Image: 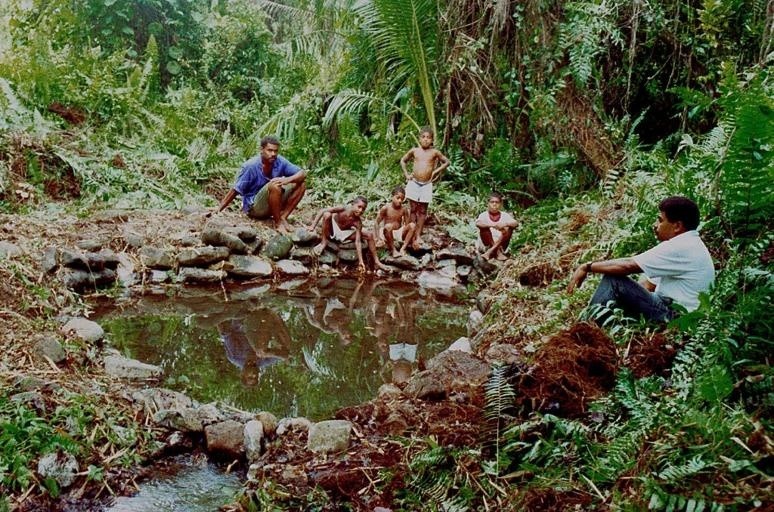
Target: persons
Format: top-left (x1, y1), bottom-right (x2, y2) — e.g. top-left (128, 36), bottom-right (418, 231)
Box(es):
top-left (365, 292), bottom-right (404, 356)
top-left (376, 186), bottom-right (416, 258)
top-left (561, 195), bottom-right (717, 336)
top-left (400, 126), bottom-right (450, 246)
top-left (379, 298), bottom-right (427, 384)
top-left (304, 274), bottom-right (365, 346)
top-left (306, 196), bottom-right (391, 273)
top-left (475, 191), bottom-right (519, 262)
top-left (216, 311), bottom-right (292, 386)
top-left (209, 135), bottom-right (306, 236)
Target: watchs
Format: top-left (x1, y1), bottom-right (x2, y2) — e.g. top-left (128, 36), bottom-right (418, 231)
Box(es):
top-left (585, 261), bottom-right (594, 274)
